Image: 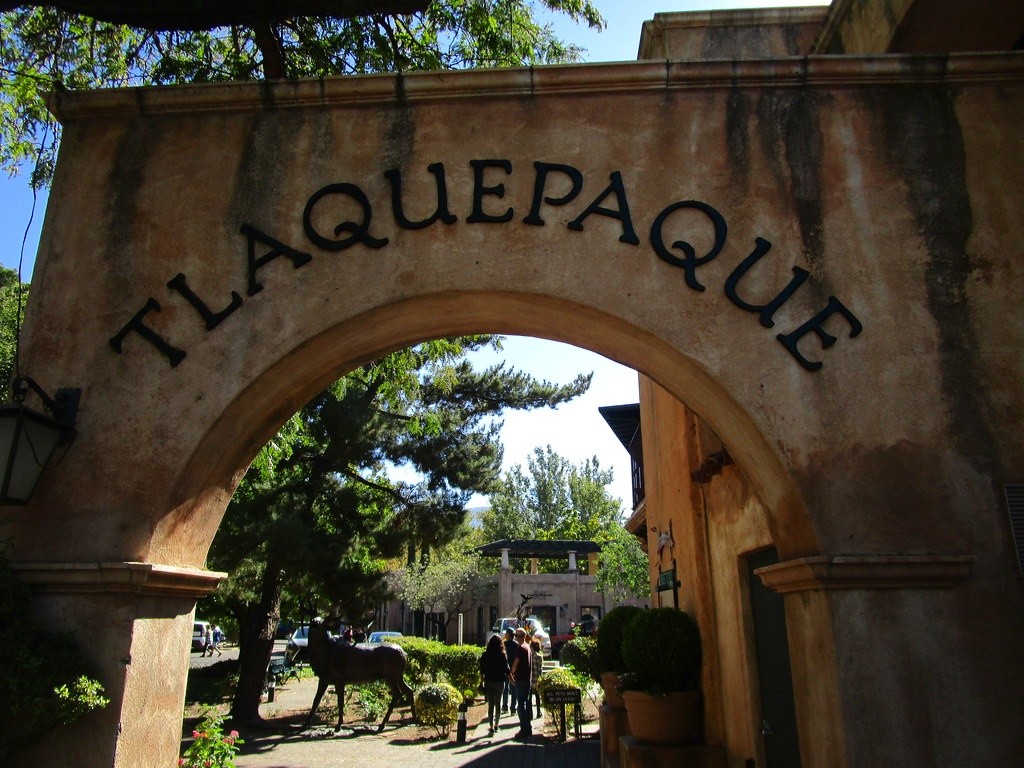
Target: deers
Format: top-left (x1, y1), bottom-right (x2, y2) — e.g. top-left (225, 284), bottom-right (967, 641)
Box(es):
top-left (298, 591), bottom-right (420, 732)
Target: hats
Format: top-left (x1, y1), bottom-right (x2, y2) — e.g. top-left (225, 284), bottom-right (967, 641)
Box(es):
top-left (507, 627), bottom-right (516, 634)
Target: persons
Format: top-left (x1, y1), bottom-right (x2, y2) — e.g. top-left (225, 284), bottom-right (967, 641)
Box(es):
top-left (343, 624), bottom-right (372, 644)
top-left (480, 627), bottom-right (543, 739)
top-left (201, 624), bottom-right (223, 658)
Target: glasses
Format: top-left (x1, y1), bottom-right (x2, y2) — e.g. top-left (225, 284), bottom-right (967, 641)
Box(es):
top-left (514, 633), bottom-right (525, 638)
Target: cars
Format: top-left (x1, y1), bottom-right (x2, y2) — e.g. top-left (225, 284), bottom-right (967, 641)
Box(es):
top-left (283, 626), bottom-right (339, 667)
top-left (192, 621), bottom-right (226, 653)
top-left (367, 630), bottom-right (403, 645)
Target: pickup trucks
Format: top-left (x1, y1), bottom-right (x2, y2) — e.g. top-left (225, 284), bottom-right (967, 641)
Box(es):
top-left (485, 618), bottom-right (552, 659)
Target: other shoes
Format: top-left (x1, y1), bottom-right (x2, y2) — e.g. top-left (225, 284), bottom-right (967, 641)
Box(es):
top-left (537, 712), bottom-right (542, 718)
top-left (494, 726), bottom-right (498, 733)
top-left (502, 705), bottom-right (508, 714)
top-left (515, 730), bottom-right (532, 740)
top-left (488, 728), bottom-right (494, 736)
top-left (510, 708), bottom-right (516, 716)
